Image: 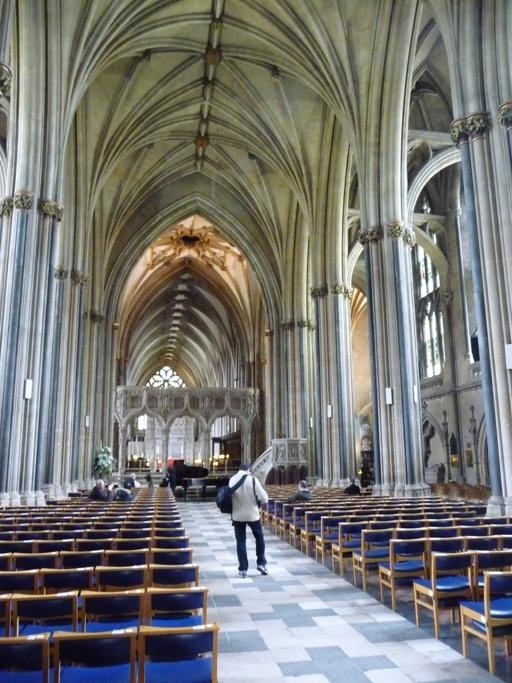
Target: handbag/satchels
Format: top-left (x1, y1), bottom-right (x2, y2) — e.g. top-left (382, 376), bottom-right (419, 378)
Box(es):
top-left (216, 474), bottom-right (248, 513)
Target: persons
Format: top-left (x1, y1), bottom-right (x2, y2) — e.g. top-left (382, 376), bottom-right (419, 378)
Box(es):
top-left (228, 464), bottom-right (269, 578)
top-left (145, 473), bottom-right (153, 488)
top-left (128, 473), bottom-right (140, 488)
top-left (89, 478), bottom-right (109, 500)
top-left (288, 480), bottom-right (312, 504)
top-left (162, 473), bottom-right (169, 487)
top-left (113, 488), bottom-right (134, 501)
top-left (344, 478), bottom-right (360, 495)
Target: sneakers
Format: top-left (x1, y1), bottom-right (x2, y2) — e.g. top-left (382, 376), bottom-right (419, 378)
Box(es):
top-left (238, 570), bottom-right (247, 578)
top-left (257, 564), bottom-right (268, 575)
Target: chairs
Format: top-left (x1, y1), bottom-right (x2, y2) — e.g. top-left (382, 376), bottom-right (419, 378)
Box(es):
top-left (498, 534), bottom-right (512, 573)
top-left (424, 536), bottom-right (465, 578)
top-left (80, 588), bottom-right (145, 631)
top-left (398, 520), bottom-right (427, 528)
top-left (428, 527), bottom-right (460, 538)
top-left (454, 518), bottom-right (482, 526)
top-left (10, 590), bottom-right (79, 637)
top-left (0, 552), bottom-right (13, 571)
top-left (149, 563), bottom-right (200, 588)
top-left (460, 569), bottom-right (512, 674)
top-left (279, 504), bottom-right (305, 542)
top-left (289, 507), bottom-right (317, 549)
top-left (413, 553), bottom-right (473, 640)
top-left (331, 521), bottom-right (369, 575)
top-left (138, 623), bottom-right (220, 683)
top-left (52, 627), bottom-right (138, 680)
top-left (32, 538), bottom-right (75, 551)
top-left (300, 510), bottom-right (329, 556)
top-left (39, 567), bottom-right (94, 593)
top-left (378, 538), bottom-right (427, 612)
top-left (0, 570), bottom-right (40, 594)
top-left (146, 587), bottom-right (208, 626)
top-left (314, 516), bottom-right (348, 565)
top-left (0, 487), bottom-right (185, 536)
top-left (96, 565), bottom-right (149, 591)
top-left (0, 541), bottom-right (32, 552)
top-left (461, 525), bottom-right (491, 536)
top-left (352, 528), bottom-right (394, 591)
top-left (151, 547), bottom-right (193, 564)
top-left (428, 519), bottom-right (453, 527)
top-left (476, 550), bottom-right (512, 601)
top-left (1, 593), bottom-right (11, 637)
top-left (395, 528), bottom-right (427, 562)
top-left (0, 634), bottom-right (49, 682)
top-left (369, 520), bottom-right (398, 529)
top-left (253, 494), bottom-right (278, 535)
top-left (76, 538), bottom-right (112, 549)
top-left (315, 493), bottom-right (487, 518)
top-left (105, 548), bottom-right (150, 565)
top-left (153, 535), bottom-right (192, 547)
top-left (482, 518), bottom-right (507, 524)
top-left (467, 536), bottom-right (500, 594)
top-left (60, 550), bottom-right (105, 568)
top-left (12, 552), bottom-right (59, 570)
top-left (491, 524), bottom-right (512, 536)
top-left (112, 536), bottom-right (153, 548)
